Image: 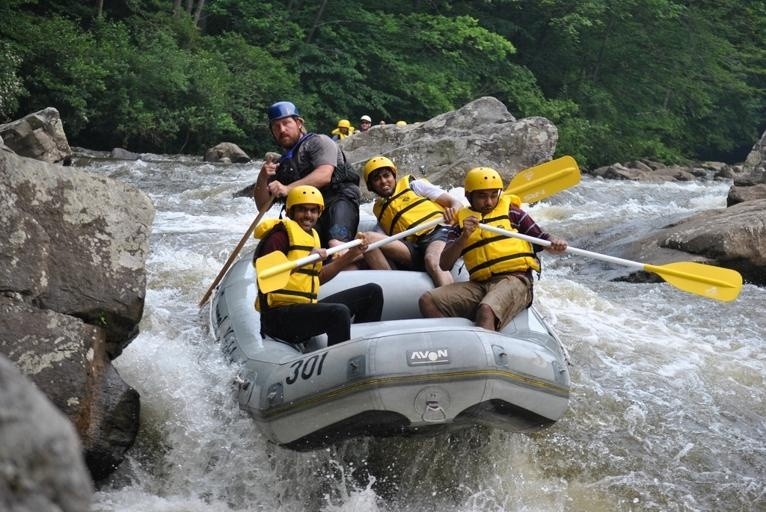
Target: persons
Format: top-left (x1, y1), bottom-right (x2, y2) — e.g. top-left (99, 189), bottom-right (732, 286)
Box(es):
top-left (252, 185), bottom-right (384, 348)
top-left (359, 156), bottom-right (464, 288)
top-left (332, 119), bottom-right (361, 141)
top-left (358, 114), bottom-right (372, 131)
top-left (254, 101), bottom-right (361, 243)
top-left (418, 167), bottom-right (567, 331)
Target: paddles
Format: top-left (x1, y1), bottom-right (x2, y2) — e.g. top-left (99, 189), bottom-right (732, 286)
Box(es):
top-left (364, 155), bottom-right (582, 254)
top-left (477, 221), bottom-right (744, 303)
top-left (255, 238), bottom-right (364, 293)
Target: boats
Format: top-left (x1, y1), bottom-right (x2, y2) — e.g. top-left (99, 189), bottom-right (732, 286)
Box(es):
top-left (209, 235), bottom-right (576, 453)
top-left (208, 201), bottom-right (571, 451)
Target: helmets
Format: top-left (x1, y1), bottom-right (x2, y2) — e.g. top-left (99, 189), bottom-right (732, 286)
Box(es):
top-left (364, 157), bottom-right (397, 195)
top-left (338, 120), bottom-right (351, 128)
top-left (360, 115), bottom-right (371, 123)
top-left (464, 167), bottom-right (503, 214)
top-left (267, 100), bottom-right (300, 122)
top-left (285, 184), bottom-right (325, 219)
